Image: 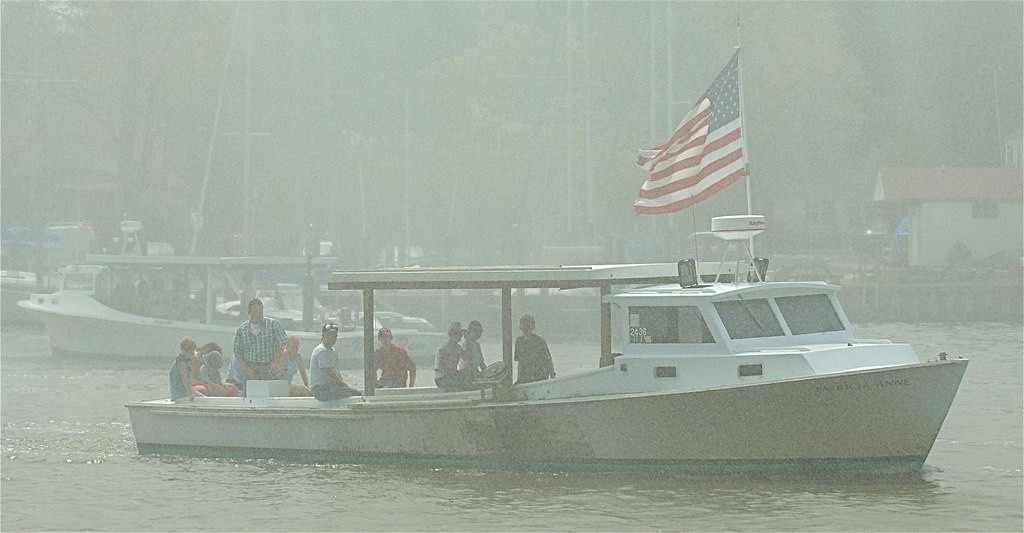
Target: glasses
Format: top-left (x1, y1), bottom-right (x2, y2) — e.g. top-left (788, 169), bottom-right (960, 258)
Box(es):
top-left (324, 324), bottom-right (338, 330)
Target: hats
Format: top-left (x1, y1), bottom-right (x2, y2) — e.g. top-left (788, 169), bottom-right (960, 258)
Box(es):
top-left (378, 328), bottom-right (392, 337)
top-left (202, 351), bottom-right (222, 369)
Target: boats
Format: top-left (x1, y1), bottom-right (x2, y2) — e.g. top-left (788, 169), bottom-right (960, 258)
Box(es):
top-left (124, 14), bottom-right (972, 483)
top-left (1, 5), bottom-right (452, 366)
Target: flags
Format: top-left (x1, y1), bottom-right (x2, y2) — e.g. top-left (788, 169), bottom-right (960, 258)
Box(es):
top-left (633, 48), bottom-right (749, 213)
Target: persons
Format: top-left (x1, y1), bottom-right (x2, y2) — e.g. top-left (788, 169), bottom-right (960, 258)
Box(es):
top-left (374, 328), bottom-right (416, 388)
top-left (311, 323), bottom-right (362, 402)
top-left (435, 320), bottom-right (486, 393)
top-left (514, 315), bottom-right (555, 384)
top-left (170, 299), bottom-right (311, 401)
